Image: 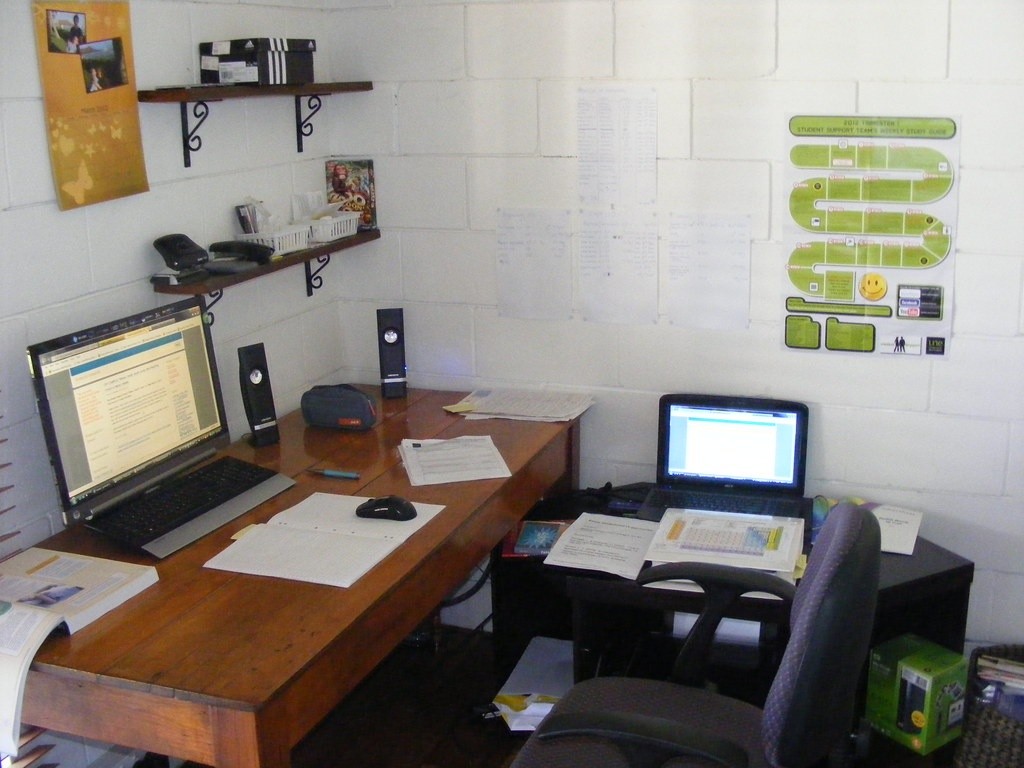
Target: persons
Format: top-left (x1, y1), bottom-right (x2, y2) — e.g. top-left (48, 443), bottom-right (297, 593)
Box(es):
top-left (65, 15), bottom-right (85, 54)
top-left (88, 66), bottom-right (110, 92)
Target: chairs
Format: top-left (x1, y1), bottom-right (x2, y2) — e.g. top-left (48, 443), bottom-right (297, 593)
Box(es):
top-left (514, 499), bottom-right (883, 768)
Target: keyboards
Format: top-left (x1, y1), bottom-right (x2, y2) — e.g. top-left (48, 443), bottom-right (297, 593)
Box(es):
top-left (82, 455), bottom-right (297, 559)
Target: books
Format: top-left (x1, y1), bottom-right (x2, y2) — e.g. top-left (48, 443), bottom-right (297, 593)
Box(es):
top-left (502, 520), bottom-right (575, 558)
top-left (977, 655), bottom-right (1024, 689)
top-left (202, 493), bottom-right (446, 588)
top-left (0, 546), bottom-right (160, 757)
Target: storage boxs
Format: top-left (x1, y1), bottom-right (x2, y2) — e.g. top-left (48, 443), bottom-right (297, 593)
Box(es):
top-left (864, 630), bottom-right (969, 756)
top-left (198, 37), bottom-right (316, 95)
top-left (326, 158), bottom-right (376, 232)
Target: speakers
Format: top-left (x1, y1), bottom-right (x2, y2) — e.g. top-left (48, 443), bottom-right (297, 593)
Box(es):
top-left (377, 307), bottom-right (407, 399)
top-left (238, 342), bottom-right (280, 449)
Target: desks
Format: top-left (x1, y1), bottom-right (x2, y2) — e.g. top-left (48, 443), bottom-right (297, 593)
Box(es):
top-left (1, 381), bottom-right (581, 768)
top-left (491, 481), bottom-right (976, 746)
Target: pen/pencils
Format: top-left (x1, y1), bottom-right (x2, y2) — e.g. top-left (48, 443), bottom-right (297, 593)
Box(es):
top-left (304, 468), bottom-right (361, 479)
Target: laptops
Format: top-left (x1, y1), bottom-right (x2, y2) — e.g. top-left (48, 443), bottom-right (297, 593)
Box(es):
top-left (635, 393), bottom-right (808, 521)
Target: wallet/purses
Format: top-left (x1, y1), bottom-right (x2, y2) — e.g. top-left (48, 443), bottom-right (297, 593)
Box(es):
top-left (301, 384), bottom-right (377, 431)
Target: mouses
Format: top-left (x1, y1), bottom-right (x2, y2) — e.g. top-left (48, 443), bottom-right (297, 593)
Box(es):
top-left (356, 494), bottom-right (418, 521)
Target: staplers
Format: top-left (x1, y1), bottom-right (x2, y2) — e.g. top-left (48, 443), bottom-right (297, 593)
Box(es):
top-left (210, 240), bottom-right (274, 265)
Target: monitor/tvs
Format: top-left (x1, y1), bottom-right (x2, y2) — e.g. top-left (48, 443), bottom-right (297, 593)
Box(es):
top-left (26, 295), bottom-right (231, 526)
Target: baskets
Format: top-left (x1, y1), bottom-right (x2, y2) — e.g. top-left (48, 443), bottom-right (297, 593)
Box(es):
top-left (951, 645), bottom-right (1024, 768)
